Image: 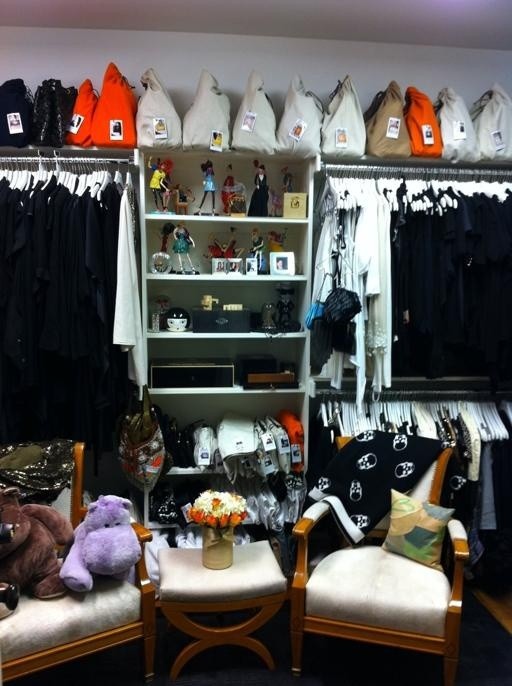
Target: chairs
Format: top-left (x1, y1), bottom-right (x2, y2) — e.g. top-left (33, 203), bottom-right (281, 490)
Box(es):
top-left (287, 435), bottom-right (470, 684)
top-left (1, 440), bottom-right (156, 683)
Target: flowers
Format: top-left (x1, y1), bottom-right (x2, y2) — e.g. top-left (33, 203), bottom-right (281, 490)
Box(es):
top-left (189, 489), bottom-right (248, 529)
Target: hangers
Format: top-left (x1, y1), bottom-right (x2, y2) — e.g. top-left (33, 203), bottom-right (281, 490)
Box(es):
top-left (0, 155), bottom-right (134, 201)
top-left (317, 384), bottom-right (512, 443)
top-left (315, 162), bottom-right (512, 213)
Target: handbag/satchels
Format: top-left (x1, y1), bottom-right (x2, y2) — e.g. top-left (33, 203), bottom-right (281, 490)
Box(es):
top-left (306, 273), bottom-right (362, 376)
top-left (64, 78), bottom-right (101, 149)
top-left (273, 73), bottom-right (324, 160)
top-left (91, 63), bottom-right (137, 149)
top-left (118, 385), bottom-right (167, 494)
top-left (469, 83), bottom-right (510, 161)
top-left (136, 68), bottom-right (182, 151)
top-left (433, 86), bottom-right (482, 162)
top-left (169, 410), bottom-right (304, 485)
top-left (231, 73), bottom-right (276, 155)
top-left (1, 78), bottom-right (35, 149)
top-left (403, 86), bottom-right (442, 158)
top-left (182, 68), bottom-right (231, 152)
top-left (364, 80), bottom-right (412, 159)
top-left (320, 75), bottom-right (366, 159)
top-left (31, 78), bottom-right (78, 149)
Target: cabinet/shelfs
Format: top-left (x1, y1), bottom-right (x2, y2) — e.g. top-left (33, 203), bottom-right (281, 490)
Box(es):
top-left (141, 151), bottom-right (315, 532)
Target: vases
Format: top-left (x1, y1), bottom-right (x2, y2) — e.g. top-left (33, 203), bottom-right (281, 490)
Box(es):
top-left (200, 528), bottom-right (234, 568)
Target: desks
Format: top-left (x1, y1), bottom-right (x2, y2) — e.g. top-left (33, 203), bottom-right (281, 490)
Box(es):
top-left (150, 543), bottom-right (286, 675)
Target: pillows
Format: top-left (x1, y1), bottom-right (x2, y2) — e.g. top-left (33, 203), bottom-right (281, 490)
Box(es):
top-left (383, 487), bottom-right (456, 573)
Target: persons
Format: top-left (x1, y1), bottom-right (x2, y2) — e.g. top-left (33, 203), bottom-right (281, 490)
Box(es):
top-left (147, 156), bottom-right (302, 333)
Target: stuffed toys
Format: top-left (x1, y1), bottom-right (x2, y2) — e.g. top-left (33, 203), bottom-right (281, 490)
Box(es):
top-left (0, 503), bottom-right (74, 619)
top-left (58, 494), bottom-right (143, 593)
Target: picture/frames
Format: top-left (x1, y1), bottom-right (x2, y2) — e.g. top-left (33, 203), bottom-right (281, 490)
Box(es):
top-left (269, 252), bottom-right (295, 275)
top-left (212, 259), bottom-right (243, 277)
